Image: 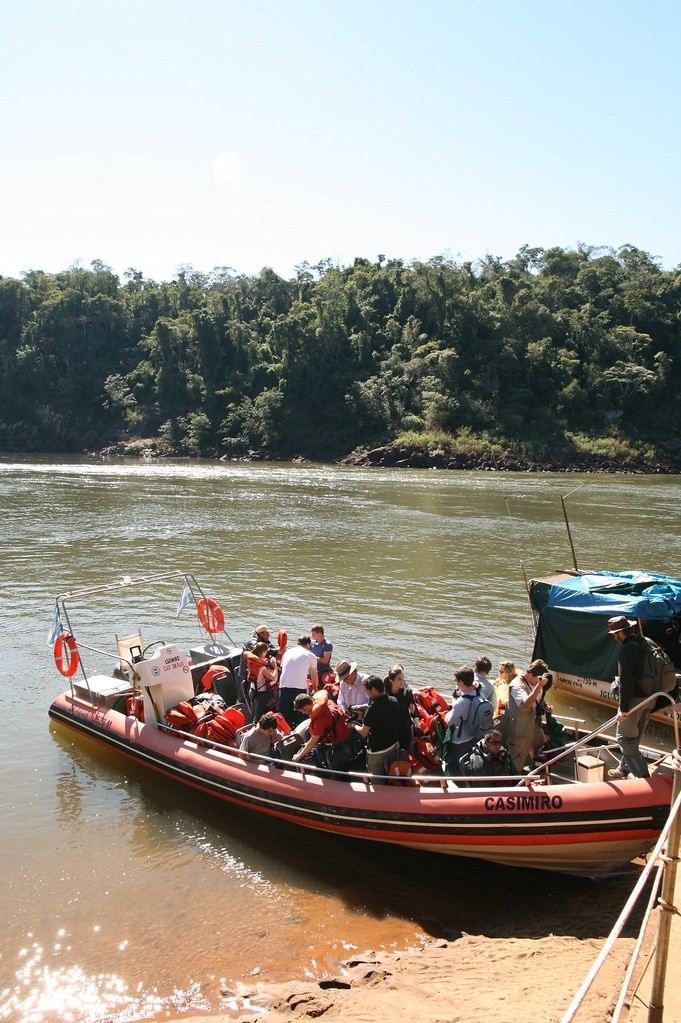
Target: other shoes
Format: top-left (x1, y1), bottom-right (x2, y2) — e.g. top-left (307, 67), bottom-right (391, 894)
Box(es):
top-left (607, 761), bottom-right (630, 780)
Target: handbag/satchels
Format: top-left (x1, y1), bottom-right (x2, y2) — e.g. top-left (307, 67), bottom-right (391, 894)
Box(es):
top-left (542, 711), bottom-right (569, 745)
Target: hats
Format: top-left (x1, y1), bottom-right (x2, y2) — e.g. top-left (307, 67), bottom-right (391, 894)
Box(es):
top-left (334, 660), bottom-right (357, 683)
top-left (255, 625), bottom-right (273, 634)
top-left (607, 616), bottom-right (638, 633)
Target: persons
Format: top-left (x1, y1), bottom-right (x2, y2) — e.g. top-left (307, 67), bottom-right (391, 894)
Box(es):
top-left (291, 693), bottom-right (353, 781)
top-left (507, 659), bottom-right (553, 775)
top-left (310, 624), bottom-right (334, 676)
top-left (247, 642), bottom-right (277, 722)
top-left (278, 634), bottom-right (318, 717)
top-left (445, 666), bottom-right (485, 775)
top-left (383, 664), bottom-right (416, 749)
top-left (237, 625), bottom-right (275, 680)
top-left (334, 659), bottom-right (370, 711)
top-left (345, 674), bottom-right (400, 786)
top-left (492, 660), bottom-right (519, 724)
top-left (460, 729), bottom-right (521, 788)
top-left (237, 713), bottom-right (278, 769)
top-left (607, 615), bottom-right (657, 778)
top-left (473, 655), bottom-right (498, 710)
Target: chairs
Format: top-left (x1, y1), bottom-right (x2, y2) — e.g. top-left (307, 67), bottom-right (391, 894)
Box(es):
top-left (241, 680), bottom-right (251, 714)
top-left (434, 710), bottom-right (449, 746)
top-left (278, 733), bottom-right (306, 771)
top-left (212, 671), bottom-right (239, 707)
top-left (226, 703), bottom-right (252, 729)
top-left (459, 753), bottom-right (473, 788)
top-left (115, 629), bottom-right (145, 681)
top-left (384, 749), bottom-right (416, 777)
top-left (235, 723), bottom-right (256, 749)
top-left (233, 666), bottom-right (242, 687)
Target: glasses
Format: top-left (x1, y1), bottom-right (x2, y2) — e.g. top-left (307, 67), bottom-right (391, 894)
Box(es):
top-left (498, 670), bottom-right (504, 674)
top-left (488, 739), bottom-right (502, 745)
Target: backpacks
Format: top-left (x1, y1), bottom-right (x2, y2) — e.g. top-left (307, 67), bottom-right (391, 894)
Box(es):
top-left (624, 636), bottom-right (677, 695)
top-left (457, 689), bottom-right (495, 739)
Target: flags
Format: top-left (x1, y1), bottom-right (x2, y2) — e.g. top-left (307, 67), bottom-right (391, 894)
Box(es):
top-left (45, 607), bottom-right (63, 649)
top-left (177, 580), bottom-right (192, 618)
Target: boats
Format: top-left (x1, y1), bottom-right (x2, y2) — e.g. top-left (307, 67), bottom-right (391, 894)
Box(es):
top-left (50, 569), bottom-right (681, 887)
top-left (502, 495), bottom-right (681, 734)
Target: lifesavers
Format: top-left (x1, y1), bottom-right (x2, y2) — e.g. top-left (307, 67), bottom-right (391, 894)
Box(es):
top-left (196, 597), bottom-right (225, 634)
top-left (53, 631), bottom-right (79, 679)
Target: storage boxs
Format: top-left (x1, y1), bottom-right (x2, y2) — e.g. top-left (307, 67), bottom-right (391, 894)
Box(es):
top-left (73, 675), bottom-right (134, 709)
top-left (573, 755), bottom-right (605, 783)
